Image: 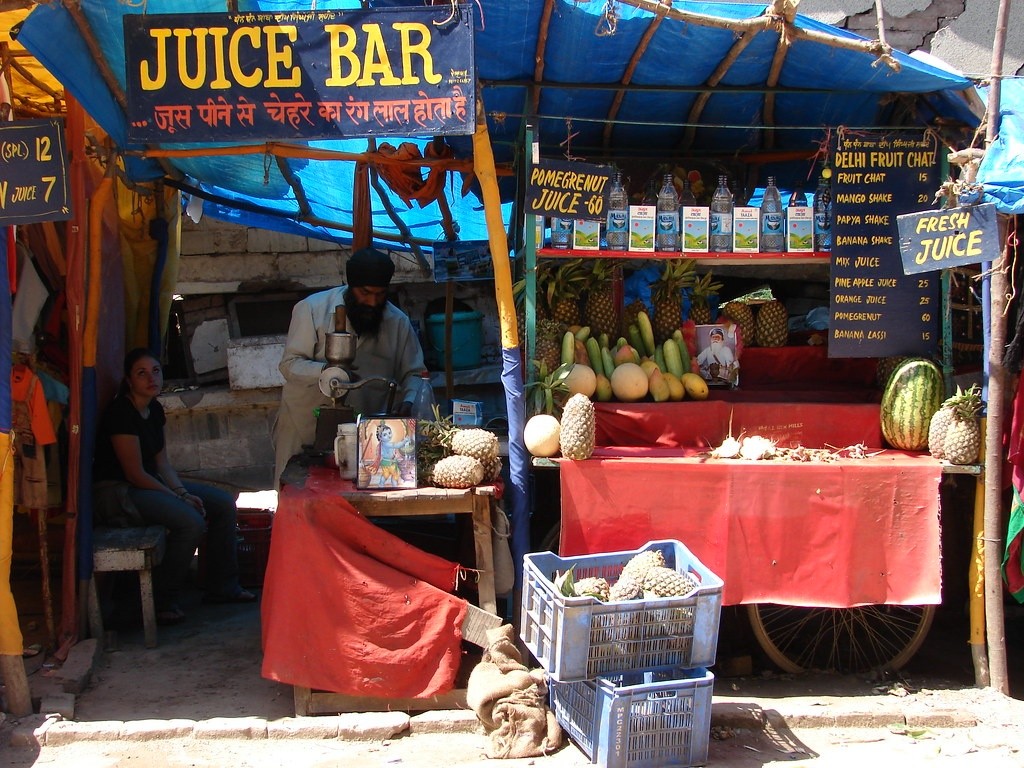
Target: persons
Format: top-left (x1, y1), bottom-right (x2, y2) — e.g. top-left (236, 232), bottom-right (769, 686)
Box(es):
top-left (695, 327), bottom-right (736, 385)
top-left (100, 347), bottom-right (257, 625)
top-left (269, 245), bottom-right (427, 494)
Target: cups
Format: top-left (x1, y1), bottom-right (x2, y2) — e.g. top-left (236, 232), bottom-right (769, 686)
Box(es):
top-left (334, 422), bottom-right (357, 479)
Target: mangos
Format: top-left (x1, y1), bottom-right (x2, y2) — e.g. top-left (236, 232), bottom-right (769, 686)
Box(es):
top-left (596, 345), bottom-right (708, 401)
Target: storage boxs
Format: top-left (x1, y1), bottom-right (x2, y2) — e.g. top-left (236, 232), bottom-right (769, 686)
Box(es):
top-left (785, 207), bottom-right (814, 252)
top-left (452, 398), bottom-right (483, 425)
top-left (226, 336), bottom-right (287, 390)
top-left (628, 205), bottom-right (656, 252)
top-left (573, 219), bottom-right (600, 250)
top-left (549, 663), bottom-right (714, 768)
top-left (519, 539), bottom-right (725, 684)
top-left (733, 207), bottom-right (761, 253)
top-left (680, 206), bottom-right (709, 253)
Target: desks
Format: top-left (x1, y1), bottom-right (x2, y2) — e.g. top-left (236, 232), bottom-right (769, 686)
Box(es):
top-left (260, 459), bottom-right (505, 717)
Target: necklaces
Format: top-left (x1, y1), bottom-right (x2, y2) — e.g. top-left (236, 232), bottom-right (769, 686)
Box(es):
top-left (129, 392), bottom-right (149, 417)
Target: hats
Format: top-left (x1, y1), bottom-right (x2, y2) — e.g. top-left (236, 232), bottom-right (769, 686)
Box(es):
top-left (345, 245), bottom-right (395, 289)
top-left (709, 327), bottom-right (725, 341)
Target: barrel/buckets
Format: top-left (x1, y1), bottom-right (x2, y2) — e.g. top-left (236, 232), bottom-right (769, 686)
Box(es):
top-left (426, 311), bottom-right (483, 369)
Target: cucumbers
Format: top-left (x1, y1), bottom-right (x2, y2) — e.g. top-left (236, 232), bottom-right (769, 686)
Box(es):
top-left (561, 311), bottom-right (690, 382)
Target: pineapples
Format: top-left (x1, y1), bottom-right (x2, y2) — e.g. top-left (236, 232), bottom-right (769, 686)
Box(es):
top-left (559, 393), bottom-right (596, 459)
top-left (873, 356), bottom-right (907, 388)
top-left (415, 403), bottom-right (502, 487)
top-left (555, 549), bottom-right (696, 660)
top-left (513, 258), bottom-right (787, 348)
top-left (928, 383), bottom-right (984, 465)
top-left (535, 318), bottom-right (561, 373)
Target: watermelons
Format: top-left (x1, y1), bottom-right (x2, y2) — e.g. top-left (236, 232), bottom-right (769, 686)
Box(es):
top-left (880, 358), bottom-right (944, 451)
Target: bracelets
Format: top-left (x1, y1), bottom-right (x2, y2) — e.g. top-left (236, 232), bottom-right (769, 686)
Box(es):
top-left (172, 486), bottom-right (189, 499)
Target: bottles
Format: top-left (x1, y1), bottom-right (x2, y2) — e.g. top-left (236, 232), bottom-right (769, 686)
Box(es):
top-left (606, 174), bottom-right (630, 251)
top-left (760, 176), bottom-right (784, 251)
top-left (813, 177), bottom-right (833, 251)
top-left (410, 371), bottom-right (437, 442)
top-left (710, 175), bottom-right (733, 252)
top-left (680, 180), bottom-right (697, 206)
top-left (657, 176), bottom-right (680, 252)
top-left (642, 179), bottom-right (659, 205)
top-left (789, 179), bottom-right (807, 206)
top-left (550, 217), bottom-right (574, 249)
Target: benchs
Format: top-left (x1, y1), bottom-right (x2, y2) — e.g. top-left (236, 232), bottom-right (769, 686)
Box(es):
top-left (88, 525), bottom-right (170, 649)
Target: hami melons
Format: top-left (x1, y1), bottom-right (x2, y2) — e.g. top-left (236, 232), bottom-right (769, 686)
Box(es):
top-left (524, 415), bottom-right (561, 457)
top-left (611, 363), bottom-right (648, 401)
top-left (558, 364), bottom-right (595, 399)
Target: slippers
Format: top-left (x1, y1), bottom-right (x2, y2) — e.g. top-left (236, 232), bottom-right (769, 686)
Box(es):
top-left (108, 598), bottom-right (186, 627)
top-left (201, 586), bottom-right (255, 604)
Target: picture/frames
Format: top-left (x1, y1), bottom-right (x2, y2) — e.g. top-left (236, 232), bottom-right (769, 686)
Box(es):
top-left (355, 414), bottom-right (417, 491)
